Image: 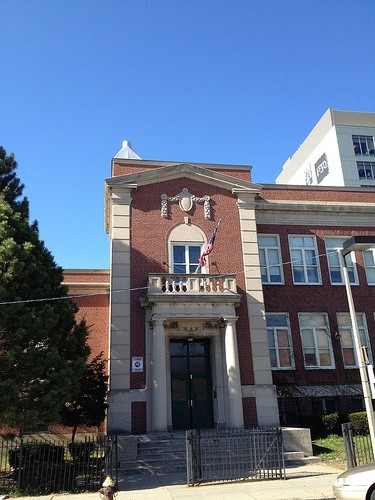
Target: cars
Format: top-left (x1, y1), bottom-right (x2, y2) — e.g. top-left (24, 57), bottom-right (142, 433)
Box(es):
top-left (332, 463), bottom-right (375, 500)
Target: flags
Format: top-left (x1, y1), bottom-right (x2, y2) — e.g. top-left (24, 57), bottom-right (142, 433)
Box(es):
top-left (198, 222), bottom-right (218, 266)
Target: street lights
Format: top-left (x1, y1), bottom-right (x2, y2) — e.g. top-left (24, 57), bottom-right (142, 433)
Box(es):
top-left (338, 234), bottom-right (375, 459)
